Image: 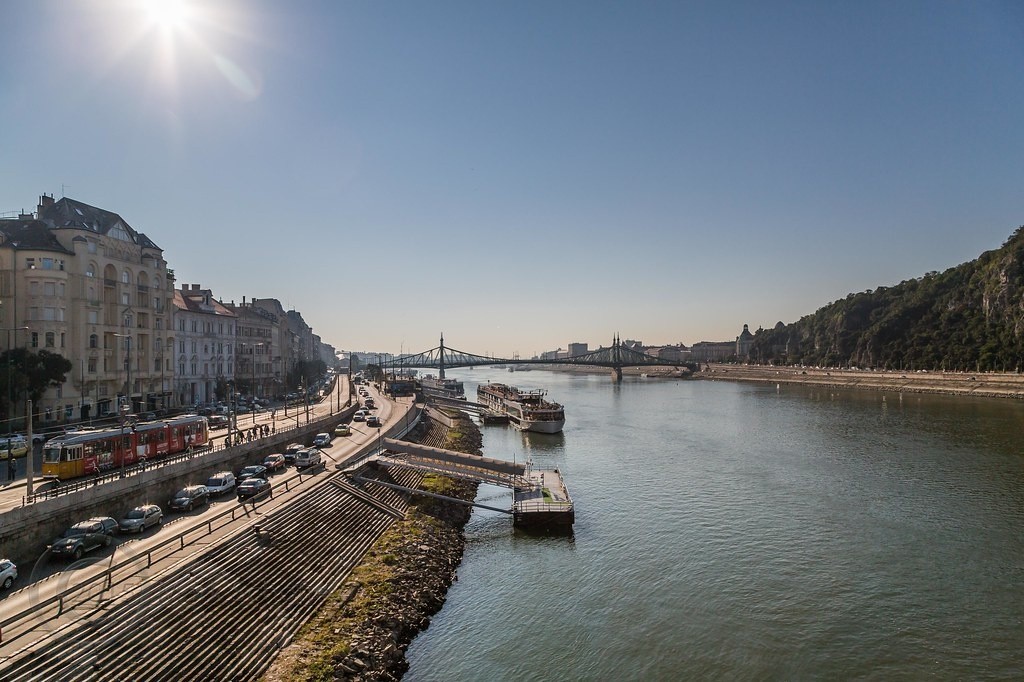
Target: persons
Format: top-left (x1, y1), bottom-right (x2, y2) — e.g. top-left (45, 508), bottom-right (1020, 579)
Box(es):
top-left (185, 444), bottom-right (193, 458)
top-left (156, 450), bottom-right (167, 466)
top-left (94, 467), bottom-right (100, 485)
top-left (11, 459), bottom-right (17, 480)
top-left (209, 439), bottom-right (213, 451)
top-left (51, 478), bottom-right (58, 496)
top-left (236, 425), bottom-right (269, 444)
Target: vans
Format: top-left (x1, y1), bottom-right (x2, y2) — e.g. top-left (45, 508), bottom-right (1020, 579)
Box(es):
top-left (206, 472), bottom-right (236, 497)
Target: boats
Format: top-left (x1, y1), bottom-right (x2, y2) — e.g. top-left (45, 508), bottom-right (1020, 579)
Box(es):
top-left (477, 379), bottom-right (566, 434)
top-left (510, 454), bottom-right (576, 529)
top-left (378, 369), bottom-right (467, 401)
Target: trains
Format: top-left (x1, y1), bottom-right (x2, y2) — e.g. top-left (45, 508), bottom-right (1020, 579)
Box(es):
top-left (42, 415), bottom-right (210, 482)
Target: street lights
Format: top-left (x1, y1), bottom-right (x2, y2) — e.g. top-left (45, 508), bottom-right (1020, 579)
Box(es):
top-left (226, 384), bottom-right (242, 447)
top-left (161, 344), bottom-right (173, 410)
top-left (334, 373), bottom-right (341, 413)
top-left (342, 351), bottom-right (352, 407)
top-left (305, 377), bottom-right (310, 423)
top-left (113, 333), bottom-right (131, 424)
top-left (118, 393), bottom-right (128, 476)
top-left (374, 353), bottom-right (412, 381)
top-left (243, 343), bottom-right (264, 423)
top-left (0, 326), bottom-right (29, 435)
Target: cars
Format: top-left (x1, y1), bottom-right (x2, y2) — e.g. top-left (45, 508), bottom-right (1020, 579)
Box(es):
top-left (0, 559), bottom-right (18, 589)
top-left (351, 376), bottom-right (370, 385)
top-left (118, 505), bottom-right (163, 533)
top-left (335, 423), bottom-right (351, 436)
top-left (186, 393), bottom-right (270, 426)
top-left (47, 516), bottom-right (119, 562)
top-left (359, 386), bottom-right (370, 397)
top-left (167, 485), bottom-right (211, 512)
top-left (280, 376), bottom-right (332, 400)
top-left (235, 465), bottom-right (271, 498)
top-left (0, 433), bottom-right (45, 460)
top-left (353, 397), bottom-right (379, 427)
top-left (263, 443), bottom-right (322, 473)
top-left (312, 433), bottom-right (331, 447)
